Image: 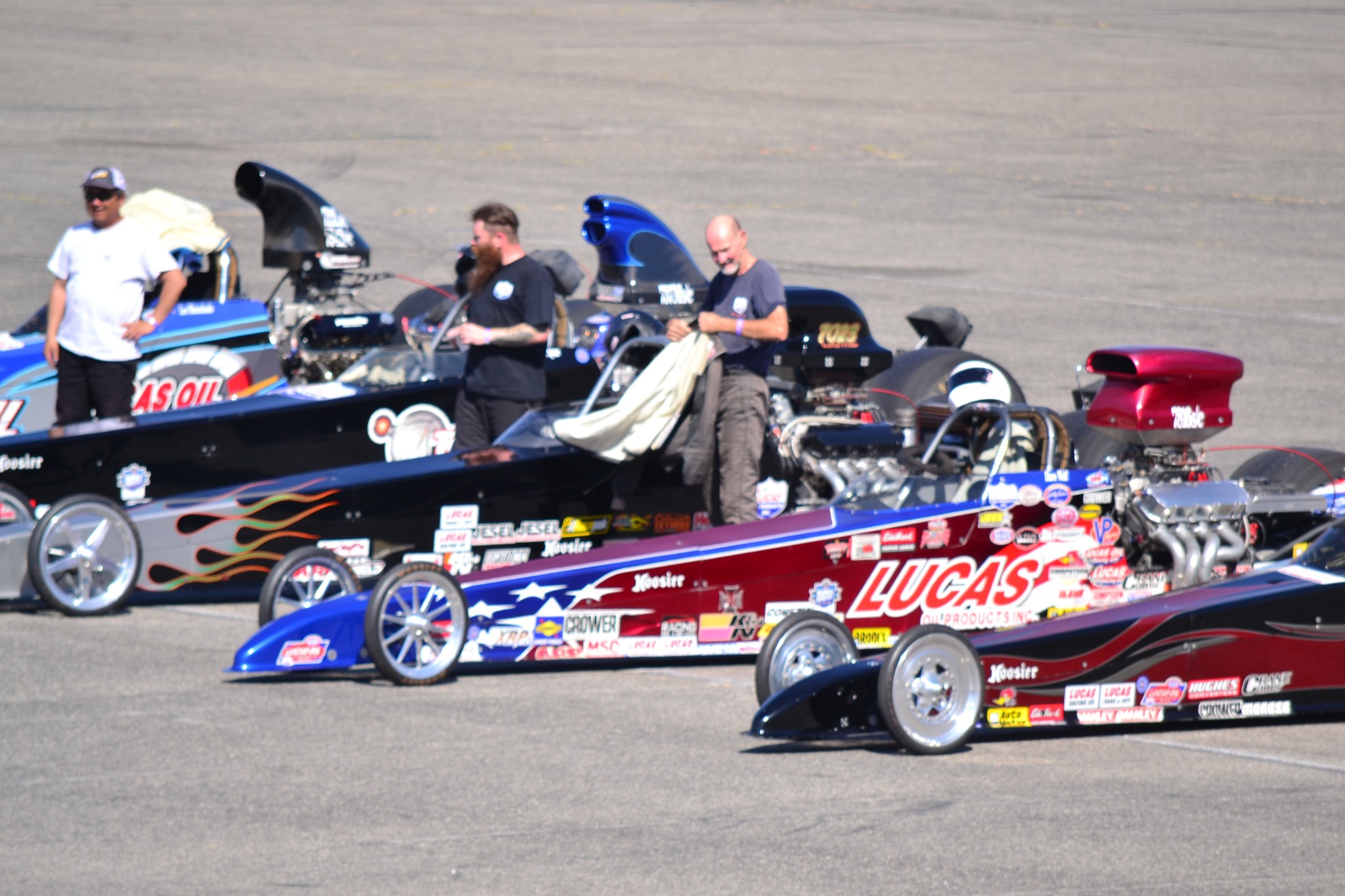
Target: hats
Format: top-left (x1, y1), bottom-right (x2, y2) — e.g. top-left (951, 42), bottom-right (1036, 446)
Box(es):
top-left (80, 166), bottom-right (127, 192)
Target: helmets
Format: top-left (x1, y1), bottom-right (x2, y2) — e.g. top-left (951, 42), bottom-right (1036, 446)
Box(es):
top-left (606, 309), bottom-right (666, 363)
top-left (947, 360), bottom-right (1014, 412)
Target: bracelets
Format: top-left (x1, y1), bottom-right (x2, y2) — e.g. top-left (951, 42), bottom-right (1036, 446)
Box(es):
top-left (483, 327), bottom-right (490, 346)
top-left (146, 315), bottom-right (160, 329)
top-left (735, 318), bottom-right (745, 336)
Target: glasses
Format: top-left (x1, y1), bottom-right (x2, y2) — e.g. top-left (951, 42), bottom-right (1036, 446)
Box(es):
top-left (85, 187), bottom-right (118, 201)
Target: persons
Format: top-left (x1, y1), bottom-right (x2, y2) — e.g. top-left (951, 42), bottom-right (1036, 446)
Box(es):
top-left (42, 164), bottom-right (188, 425)
top-left (663, 214), bottom-right (789, 528)
top-left (944, 360), bottom-right (1033, 506)
top-left (444, 202), bottom-right (554, 452)
